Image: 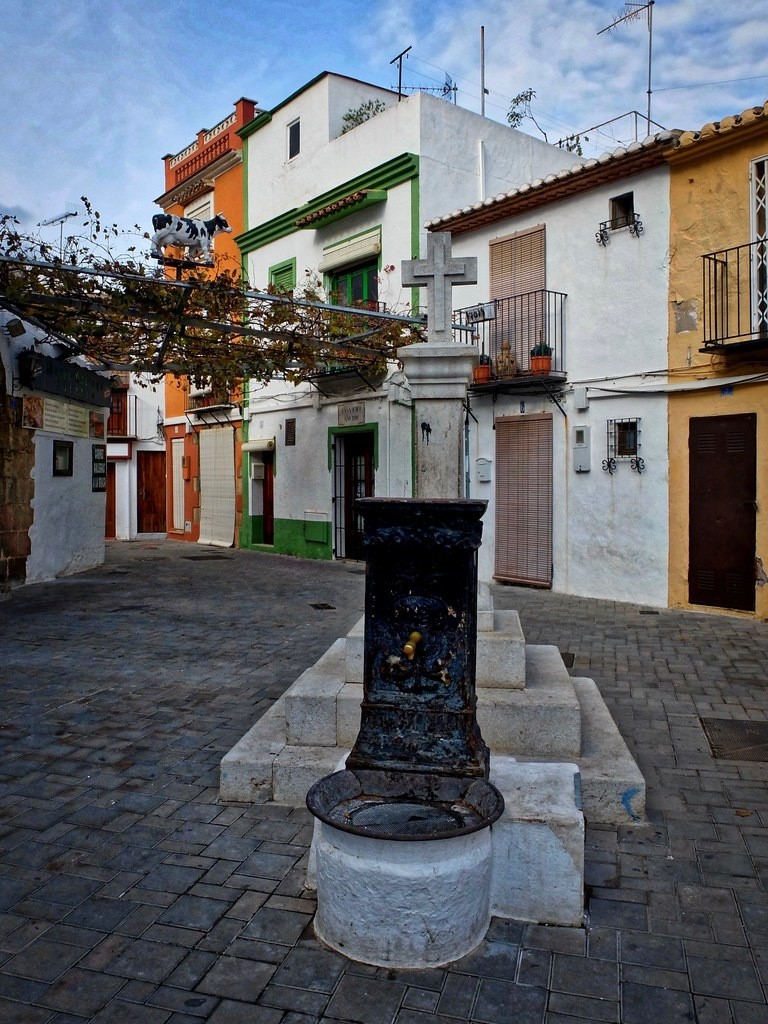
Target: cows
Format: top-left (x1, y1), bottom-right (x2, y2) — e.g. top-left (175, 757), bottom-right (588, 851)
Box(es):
top-left (150, 212), bottom-right (233, 266)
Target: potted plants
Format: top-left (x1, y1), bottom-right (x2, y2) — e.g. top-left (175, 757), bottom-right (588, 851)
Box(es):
top-left (474, 355), bottom-right (493, 384)
top-left (531, 343), bottom-right (554, 376)
